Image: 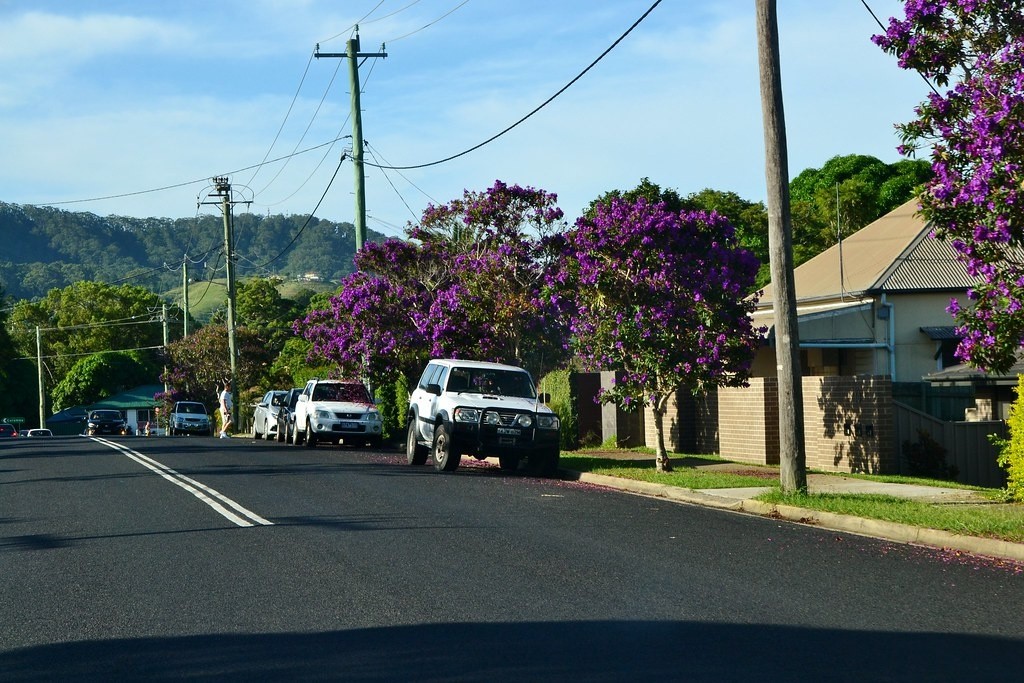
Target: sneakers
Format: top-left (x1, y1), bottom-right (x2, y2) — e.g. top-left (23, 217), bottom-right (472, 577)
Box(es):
top-left (218, 430), bottom-right (230, 439)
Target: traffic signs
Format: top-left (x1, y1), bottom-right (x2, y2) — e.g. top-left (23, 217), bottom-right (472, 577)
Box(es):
top-left (1, 417), bottom-right (25, 423)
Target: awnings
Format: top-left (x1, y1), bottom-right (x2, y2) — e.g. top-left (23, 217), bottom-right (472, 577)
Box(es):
top-left (739, 301), bottom-right (876, 343)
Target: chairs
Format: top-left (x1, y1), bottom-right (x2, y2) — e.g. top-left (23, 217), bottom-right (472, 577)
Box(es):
top-left (448, 375), bottom-right (467, 389)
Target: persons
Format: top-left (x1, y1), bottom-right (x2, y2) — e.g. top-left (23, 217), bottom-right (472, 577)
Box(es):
top-left (273, 397), bottom-right (282, 406)
top-left (219, 384), bottom-right (234, 438)
top-left (145, 422), bottom-right (151, 436)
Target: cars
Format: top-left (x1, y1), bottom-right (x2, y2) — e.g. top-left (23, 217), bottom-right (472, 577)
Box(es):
top-left (19, 430), bottom-right (29, 437)
top-left (276, 388), bottom-right (306, 443)
top-left (169, 401), bottom-right (210, 436)
top-left (86, 410), bottom-right (126, 435)
top-left (252, 391), bottom-right (288, 441)
top-left (27, 429), bottom-right (53, 437)
top-left (0, 424), bottom-right (18, 437)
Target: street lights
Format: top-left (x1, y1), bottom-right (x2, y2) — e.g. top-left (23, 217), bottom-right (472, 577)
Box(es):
top-left (188, 278), bottom-right (241, 433)
top-left (132, 310), bottom-right (170, 437)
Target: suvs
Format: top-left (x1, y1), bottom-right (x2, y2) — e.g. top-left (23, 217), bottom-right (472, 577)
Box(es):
top-left (406, 357), bottom-right (560, 474)
top-left (292, 377), bottom-right (383, 448)
